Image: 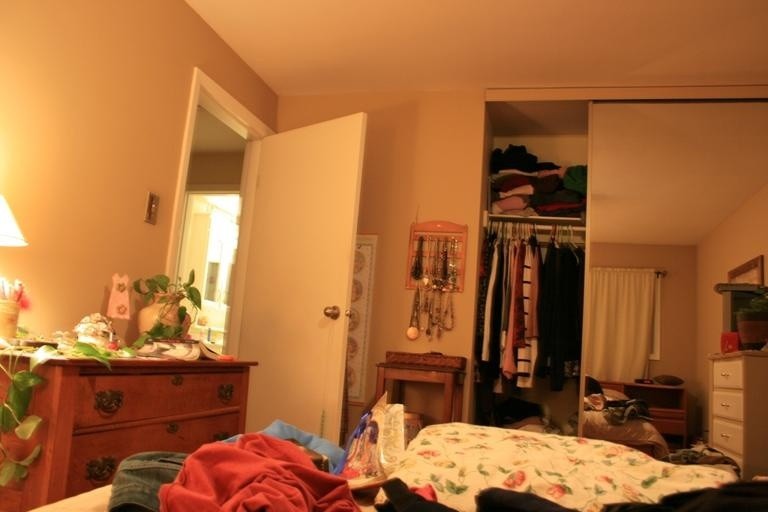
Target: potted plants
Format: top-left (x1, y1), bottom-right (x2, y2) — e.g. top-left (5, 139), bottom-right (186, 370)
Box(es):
top-left (0, 269), bottom-right (202, 488)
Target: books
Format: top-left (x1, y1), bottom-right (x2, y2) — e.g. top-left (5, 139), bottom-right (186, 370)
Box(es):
top-left (136, 336), bottom-right (225, 365)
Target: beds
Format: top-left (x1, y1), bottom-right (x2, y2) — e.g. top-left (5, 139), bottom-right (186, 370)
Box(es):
top-left (25, 436), bottom-right (768, 511)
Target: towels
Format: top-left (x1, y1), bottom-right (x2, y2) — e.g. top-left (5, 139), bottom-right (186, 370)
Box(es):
top-left (584, 268), bottom-right (659, 384)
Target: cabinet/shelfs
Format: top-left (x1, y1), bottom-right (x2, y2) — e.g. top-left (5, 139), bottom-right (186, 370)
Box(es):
top-left (706, 349), bottom-right (768, 482)
top-left (0, 346), bottom-right (258, 512)
top-left (618, 380), bottom-right (689, 442)
top-left (465, 87), bottom-right (590, 437)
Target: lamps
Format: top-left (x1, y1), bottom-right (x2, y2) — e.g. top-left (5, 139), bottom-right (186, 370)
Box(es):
top-left (0, 192), bottom-right (29, 247)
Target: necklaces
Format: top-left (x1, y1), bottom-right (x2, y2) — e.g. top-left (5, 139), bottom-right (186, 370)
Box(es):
top-left (404, 234), bottom-right (460, 343)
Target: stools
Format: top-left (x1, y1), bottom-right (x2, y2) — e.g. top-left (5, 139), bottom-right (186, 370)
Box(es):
top-left (374, 359), bottom-right (467, 424)
